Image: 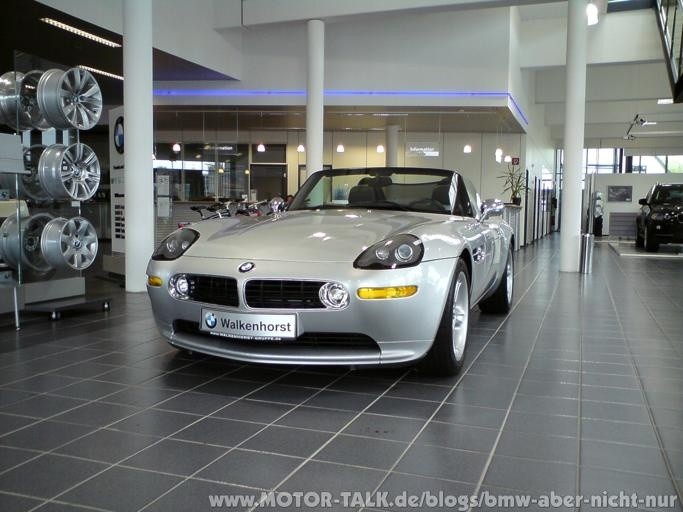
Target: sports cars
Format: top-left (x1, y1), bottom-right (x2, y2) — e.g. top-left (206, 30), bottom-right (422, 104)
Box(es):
top-left (138, 162), bottom-right (519, 387)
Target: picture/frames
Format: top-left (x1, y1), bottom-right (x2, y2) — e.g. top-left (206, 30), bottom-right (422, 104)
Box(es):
top-left (606, 186), bottom-right (632, 202)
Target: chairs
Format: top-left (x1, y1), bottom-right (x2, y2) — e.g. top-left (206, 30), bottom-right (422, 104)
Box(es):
top-left (347, 176), bottom-right (461, 212)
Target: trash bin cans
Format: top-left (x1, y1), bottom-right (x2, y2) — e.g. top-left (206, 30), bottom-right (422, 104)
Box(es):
top-left (581, 233), bottom-right (594, 274)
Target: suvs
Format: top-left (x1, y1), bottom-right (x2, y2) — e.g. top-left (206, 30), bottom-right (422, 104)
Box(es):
top-left (631, 181), bottom-right (682, 253)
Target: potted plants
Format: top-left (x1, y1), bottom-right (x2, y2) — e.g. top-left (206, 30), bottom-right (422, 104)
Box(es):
top-left (496, 163), bottom-right (532, 206)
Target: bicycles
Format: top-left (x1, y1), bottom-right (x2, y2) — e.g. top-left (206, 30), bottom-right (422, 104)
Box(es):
top-left (179, 196), bottom-right (268, 227)
